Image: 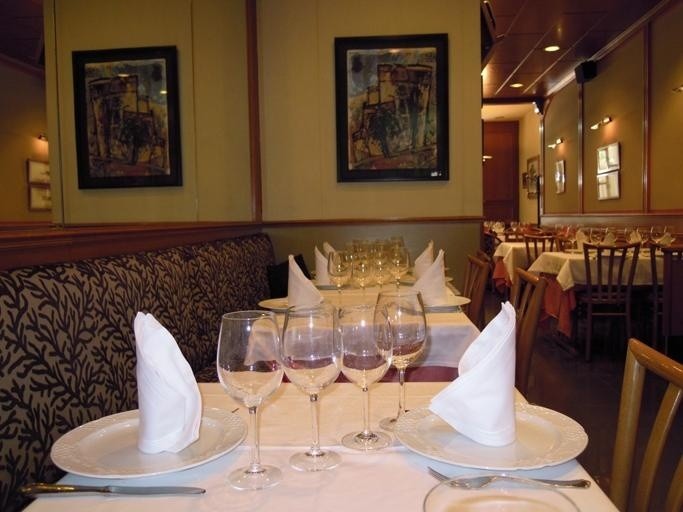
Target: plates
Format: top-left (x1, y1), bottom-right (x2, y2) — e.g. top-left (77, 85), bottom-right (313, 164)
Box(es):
top-left (48, 406), bottom-right (247, 479)
top-left (394, 403), bottom-right (589, 511)
top-left (309, 265), bottom-right (470, 309)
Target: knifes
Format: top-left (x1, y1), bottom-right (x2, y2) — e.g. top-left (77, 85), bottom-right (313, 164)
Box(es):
top-left (19, 484), bottom-right (208, 495)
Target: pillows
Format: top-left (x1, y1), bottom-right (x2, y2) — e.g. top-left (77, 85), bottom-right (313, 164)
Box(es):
top-left (268, 252), bottom-right (309, 298)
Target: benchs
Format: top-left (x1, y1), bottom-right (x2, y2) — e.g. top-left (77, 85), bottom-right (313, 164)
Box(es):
top-left (0, 234), bottom-right (277, 512)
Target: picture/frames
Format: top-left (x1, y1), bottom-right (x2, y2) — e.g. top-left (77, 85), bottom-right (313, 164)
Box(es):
top-left (597, 141), bottom-right (619, 174)
top-left (597, 170), bottom-right (619, 199)
top-left (28, 186), bottom-right (52, 211)
top-left (555, 159), bottom-right (565, 194)
top-left (26, 158), bottom-right (51, 185)
top-left (72, 45), bottom-right (183, 190)
top-left (526, 155), bottom-right (541, 199)
top-left (334, 32), bottom-right (449, 181)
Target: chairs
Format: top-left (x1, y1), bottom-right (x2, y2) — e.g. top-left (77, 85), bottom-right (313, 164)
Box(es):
top-left (462, 254), bottom-right (486, 328)
top-left (494, 269), bottom-right (549, 387)
top-left (611, 336), bottom-right (682, 512)
top-left (482, 221), bottom-right (682, 369)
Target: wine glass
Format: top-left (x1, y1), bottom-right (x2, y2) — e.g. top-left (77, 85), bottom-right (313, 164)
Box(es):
top-left (372, 289), bottom-right (427, 431)
top-left (214, 309), bottom-right (284, 492)
top-left (566, 223), bottom-right (677, 257)
top-left (483, 219), bottom-right (531, 238)
top-left (332, 307), bottom-right (392, 455)
top-left (284, 304), bottom-right (344, 474)
top-left (326, 235), bottom-right (415, 312)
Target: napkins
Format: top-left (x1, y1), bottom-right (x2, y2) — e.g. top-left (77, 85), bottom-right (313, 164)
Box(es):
top-left (431, 299), bottom-right (517, 448)
top-left (134, 309), bottom-right (203, 454)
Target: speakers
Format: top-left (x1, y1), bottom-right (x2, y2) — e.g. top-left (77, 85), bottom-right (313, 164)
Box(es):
top-left (574, 60), bottom-right (597, 82)
top-left (534, 97), bottom-right (544, 114)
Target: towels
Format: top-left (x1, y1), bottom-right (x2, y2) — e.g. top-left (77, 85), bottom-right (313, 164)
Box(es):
top-left (313, 246), bottom-right (339, 285)
top-left (321, 242), bottom-right (338, 259)
top-left (414, 239), bottom-right (435, 277)
top-left (287, 254), bottom-right (324, 306)
top-left (412, 250), bottom-right (449, 305)
